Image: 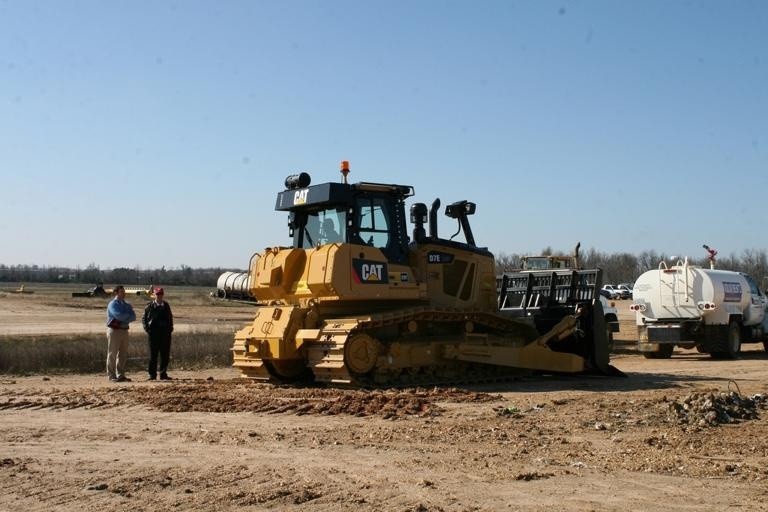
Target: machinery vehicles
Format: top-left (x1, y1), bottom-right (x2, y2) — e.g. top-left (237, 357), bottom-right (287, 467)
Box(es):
top-left (631, 245), bottom-right (768, 360)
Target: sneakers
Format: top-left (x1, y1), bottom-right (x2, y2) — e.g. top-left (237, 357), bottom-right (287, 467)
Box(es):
top-left (148, 377), bottom-right (156, 380)
top-left (160, 377), bottom-right (172, 380)
top-left (119, 377), bottom-right (132, 382)
top-left (112, 377), bottom-right (118, 381)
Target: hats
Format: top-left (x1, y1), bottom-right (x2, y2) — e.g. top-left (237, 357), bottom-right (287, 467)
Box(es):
top-left (154, 288), bottom-right (163, 294)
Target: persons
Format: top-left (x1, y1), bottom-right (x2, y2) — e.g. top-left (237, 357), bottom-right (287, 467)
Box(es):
top-left (105, 284), bottom-right (135, 381)
top-left (143, 287), bottom-right (174, 380)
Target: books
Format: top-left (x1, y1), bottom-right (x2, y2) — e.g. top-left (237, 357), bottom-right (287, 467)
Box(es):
top-left (108, 319), bottom-right (120, 331)
